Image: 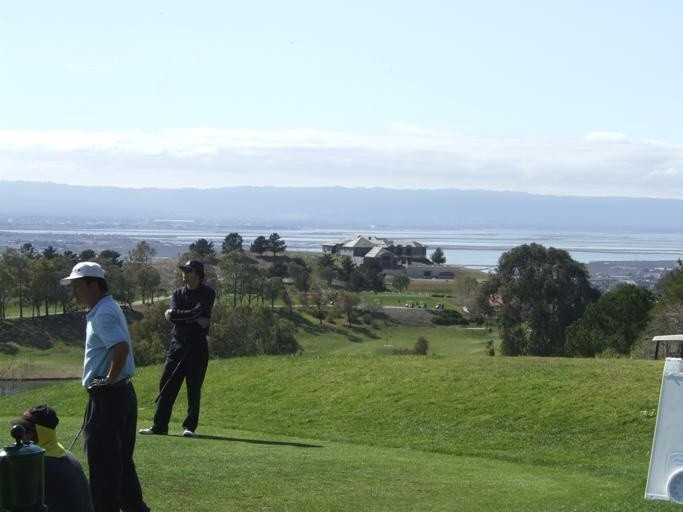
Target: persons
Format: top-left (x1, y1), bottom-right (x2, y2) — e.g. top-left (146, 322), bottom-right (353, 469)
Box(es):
top-left (11, 405), bottom-right (90, 512)
top-left (398, 298), bottom-right (447, 310)
top-left (59, 262), bottom-right (149, 512)
top-left (137, 259), bottom-right (216, 436)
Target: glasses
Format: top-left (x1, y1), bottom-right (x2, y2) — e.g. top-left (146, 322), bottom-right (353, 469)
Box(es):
top-left (182, 268), bottom-right (192, 273)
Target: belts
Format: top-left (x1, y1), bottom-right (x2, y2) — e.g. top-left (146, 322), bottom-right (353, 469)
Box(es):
top-left (94, 378), bottom-right (130, 392)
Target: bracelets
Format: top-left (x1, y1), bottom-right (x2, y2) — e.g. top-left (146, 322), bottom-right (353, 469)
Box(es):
top-left (106, 375), bottom-right (115, 384)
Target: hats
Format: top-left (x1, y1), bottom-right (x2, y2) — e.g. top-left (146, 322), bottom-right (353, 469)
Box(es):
top-left (59, 261), bottom-right (106, 287)
top-left (179, 260), bottom-right (204, 274)
top-left (11, 403), bottom-right (60, 429)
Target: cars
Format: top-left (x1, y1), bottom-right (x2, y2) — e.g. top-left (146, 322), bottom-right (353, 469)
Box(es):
top-left (417, 303), bottom-right (427, 308)
top-left (404, 301), bottom-right (413, 307)
top-left (329, 301), bottom-right (336, 305)
top-left (434, 304), bottom-right (444, 310)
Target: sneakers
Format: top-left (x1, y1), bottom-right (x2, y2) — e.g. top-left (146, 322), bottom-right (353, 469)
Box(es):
top-left (138, 427), bottom-right (168, 435)
top-left (183, 428), bottom-right (194, 436)
